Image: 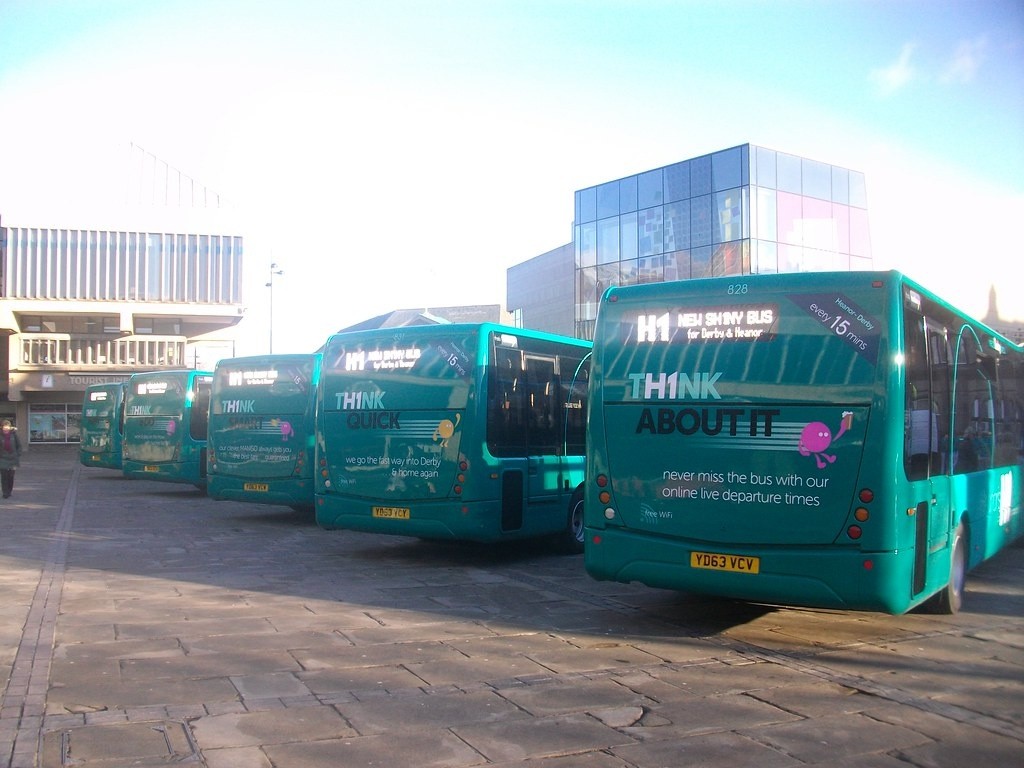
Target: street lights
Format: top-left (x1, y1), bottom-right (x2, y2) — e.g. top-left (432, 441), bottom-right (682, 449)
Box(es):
top-left (267, 263), bottom-right (284, 355)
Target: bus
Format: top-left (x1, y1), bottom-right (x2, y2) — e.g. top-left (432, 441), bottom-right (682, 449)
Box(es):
top-left (78, 382), bottom-right (126, 469)
top-left (315, 322), bottom-right (594, 554)
top-left (583, 268), bottom-right (1024, 616)
top-left (121, 371), bottom-right (214, 490)
top-left (206, 353), bottom-right (324, 516)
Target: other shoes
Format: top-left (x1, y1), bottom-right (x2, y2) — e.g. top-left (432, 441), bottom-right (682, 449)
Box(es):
top-left (2, 491), bottom-right (9, 498)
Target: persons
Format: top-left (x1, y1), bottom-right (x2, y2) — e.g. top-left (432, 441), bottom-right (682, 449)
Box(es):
top-left (0, 420), bottom-right (22, 498)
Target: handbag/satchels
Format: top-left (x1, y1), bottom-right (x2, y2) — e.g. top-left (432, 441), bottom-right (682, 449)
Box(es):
top-left (13, 431), bottom-right (23, 456)
